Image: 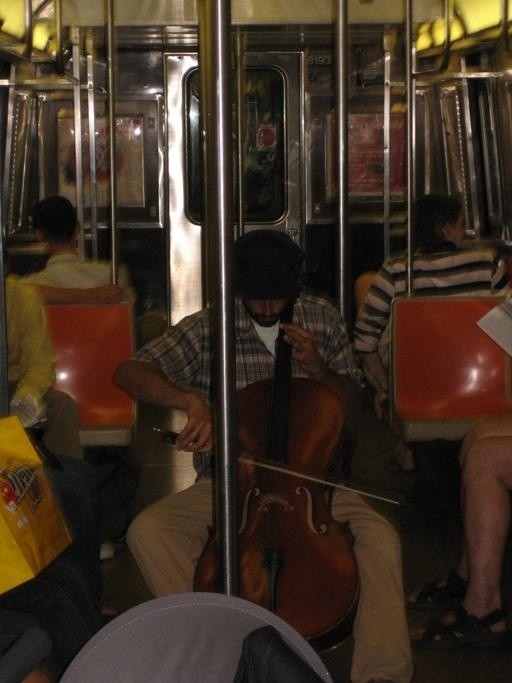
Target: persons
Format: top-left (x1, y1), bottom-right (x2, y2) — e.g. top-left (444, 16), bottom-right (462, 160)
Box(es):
top-left (406, 410), bottom-right (512, 649)
top-left (19, 195), bottom-right (138, 308)
top-left (1, 253), bottom-right (100, 679)
top-left (112, 231), bottom-right (416, 683)
top-left (354, 195), bottom-right (509, 472)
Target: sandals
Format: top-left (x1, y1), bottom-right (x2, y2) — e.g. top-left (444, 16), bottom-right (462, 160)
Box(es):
top-left (403, 566), bottom-right (467, 610)
top-left (411, 602), bottom-right (512, 651)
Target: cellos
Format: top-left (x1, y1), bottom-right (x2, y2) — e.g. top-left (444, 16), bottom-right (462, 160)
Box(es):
top-left (192, 299), bottom-right (360, 657)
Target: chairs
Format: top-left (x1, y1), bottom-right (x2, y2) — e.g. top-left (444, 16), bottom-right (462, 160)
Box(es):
top-left (379, 294), bottom-right (511, 443)
top-left (28, 293), bottom-right (142, 443)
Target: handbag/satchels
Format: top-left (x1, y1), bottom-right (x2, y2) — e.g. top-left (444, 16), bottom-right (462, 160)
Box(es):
top-left (0, 416), bottom-right (73, 596)
